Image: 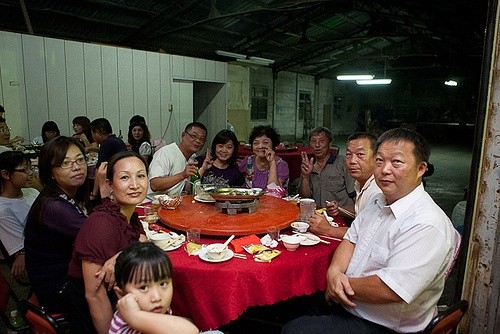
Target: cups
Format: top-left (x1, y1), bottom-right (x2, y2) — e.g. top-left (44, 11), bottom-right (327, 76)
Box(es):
top-left (186, 228), bottom-right (200, 245)
top-left (266, 226), bottom-right (280, 241)
top-left (144, 205), bottom-right (154, 216)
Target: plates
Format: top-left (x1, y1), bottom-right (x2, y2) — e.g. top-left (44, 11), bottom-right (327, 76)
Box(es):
top-left (210, 187), bottom-right (263, 198)
top-left (194, 194), bottom-right (215, 202)
top-left (162, 240), bottom-right (182, 251)
top-left (199, 247), bottom-right (234, 262)
top-left (292, 234), bottom-right (320, 246)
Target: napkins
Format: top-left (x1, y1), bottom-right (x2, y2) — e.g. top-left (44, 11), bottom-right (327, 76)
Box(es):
top-left (260, 234), bottom-right (278, 247)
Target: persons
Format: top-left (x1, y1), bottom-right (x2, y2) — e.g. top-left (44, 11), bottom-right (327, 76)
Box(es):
top-left (72, 116), bottom-right (99, 152)
top-left (0, 105), bottom-right (22, 152)
top-left (238, 125), bottom-right (289, 196)
top-left (281, 128), bottom-right (456, 334)
top-left (148, 122), bottom-right (208, 196)
top-left (83, 118), bottom-right (127, 211)
top-left (38, 121), bottom-right (60, 144)
top-left (309, 132), bottom-right (383, 239)
top-left (24, 135), bottom-right (90, 314)
top-left (0, 151), bottom-right (40, 277)
top-left (61, 150), bottom-right (149, 334)
top-left (129, 116), bottom-right (151, 149)
top-left (194, 129), bottom-right (241, 187)
top-left (107, 242), bottom-right (199, 334)
top-left (298, 126), bottom-right (356, 207)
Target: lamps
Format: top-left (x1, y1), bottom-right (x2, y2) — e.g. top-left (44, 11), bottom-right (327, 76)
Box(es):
top-left (336, 52), bottom-right (392, 85)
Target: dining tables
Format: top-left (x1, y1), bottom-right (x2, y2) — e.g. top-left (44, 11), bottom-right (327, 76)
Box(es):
top-left (235, 143), bottom-right (315, 192)
top-left (130, 195), bottom-right (349, 330)
top-left (30, 152), bottom-right (99, 191)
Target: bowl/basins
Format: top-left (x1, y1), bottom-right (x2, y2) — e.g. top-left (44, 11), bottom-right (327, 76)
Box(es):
top-left (158, 195), bottom-right (183, 209)
top-left (282, 236), bottom-right (300, 251)
top-left (291, 222), bottom-right (310, 231)
top-left (151, 233), bottom-right (169, 248)
top-left (206, 243), bottom-right (228, 260)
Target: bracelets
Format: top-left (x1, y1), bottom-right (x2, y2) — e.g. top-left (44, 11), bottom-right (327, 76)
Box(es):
top-left (90, 192), bottom-right (96, 197)
top-left (20, 251), bottom-right (26, 255)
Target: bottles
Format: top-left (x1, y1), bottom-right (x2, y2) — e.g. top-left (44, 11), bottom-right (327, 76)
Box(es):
top-left (300, 198), bottom-right (315, 224)
top-left (191, 160), bottom-right (201, 194)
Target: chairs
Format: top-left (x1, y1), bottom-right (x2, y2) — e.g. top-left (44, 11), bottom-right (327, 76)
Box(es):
top-left (0, 138), bottom-right (469, 334)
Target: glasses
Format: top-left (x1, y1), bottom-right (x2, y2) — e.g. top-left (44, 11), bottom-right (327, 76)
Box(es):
top-left (10, 167), bottom-right (35, 174)
top-left (185, 131), bottom-right (207, 143)
top-left (51, 156), bottom-right (89, 169)
top-left (0, 127), bottom-right (12, 134)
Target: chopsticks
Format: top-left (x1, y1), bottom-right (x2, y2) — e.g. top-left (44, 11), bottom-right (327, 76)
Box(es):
top-left (233, 253), bottom-right (247, 259)
top-left (326, 201), bottom-right (355, 218)
